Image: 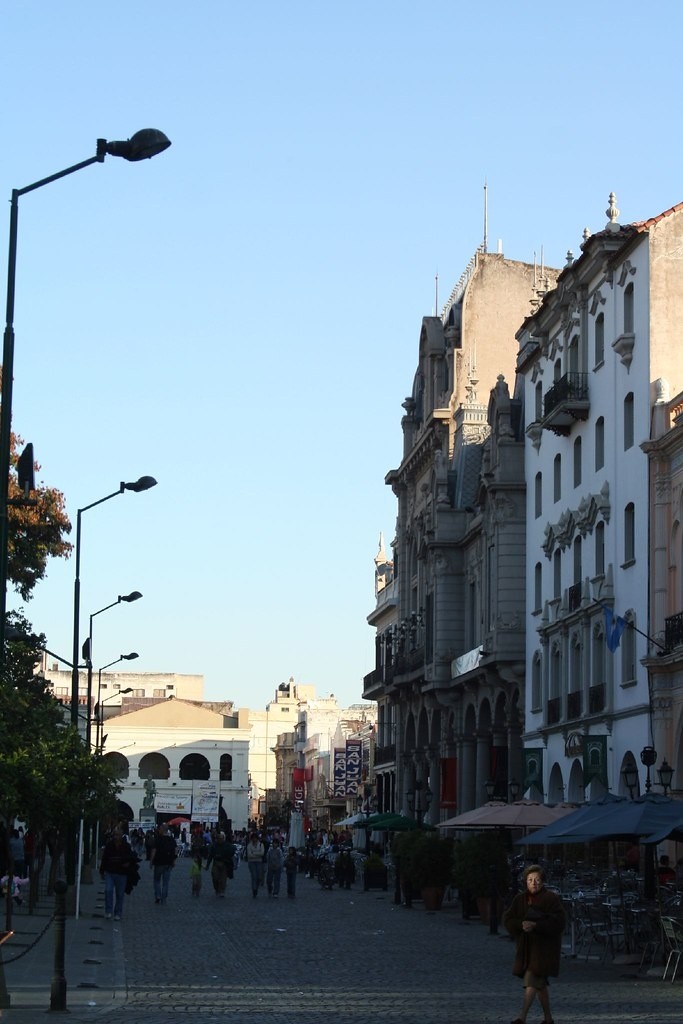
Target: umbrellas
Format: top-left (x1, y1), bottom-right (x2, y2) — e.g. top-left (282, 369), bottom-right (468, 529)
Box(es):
top-left (167, 817), bottom-right (192, 825)
top-left (517, 792), bottom-right (683, 951)
top-left (334, 810), bottom-right (432, 832)
top-left (438, 801), bottom-right (579, 827)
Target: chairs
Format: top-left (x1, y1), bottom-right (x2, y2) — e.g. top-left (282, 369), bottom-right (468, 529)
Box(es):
top-left (513, 854), bottom-right (683, 984)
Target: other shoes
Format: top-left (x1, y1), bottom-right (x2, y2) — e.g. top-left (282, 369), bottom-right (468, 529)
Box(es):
top-left (288, 895), bottom-right (295, 899)
top-left (274, 894), bottom-right (278, 898)
top-left (196, 892), bottom-right (199, 897)
top-left (106, 912), bottom-right (112, 919)
top-left (155, 898), bottom-right (160, 903)
top-left (113, 915), bottom-right (120, 920)
top-left (220, 893), bottom-right (224, 897)
top-left (215, 890), bottom-right (218, 896)
top-left (253, 890), bottom-right (257, 897)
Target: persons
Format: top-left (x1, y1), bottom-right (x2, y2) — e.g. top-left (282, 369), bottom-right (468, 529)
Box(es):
top-left (657, 854), bottom-right (677, 883)
top-left (506, 864), bottom-right (560, 1024)
top-left (0, 872), bottom-right (29, 906)
top-left (0, 819), bottom-right (353, 920)
top-left (144, 774), bottom-right (158, 812)
top-left (547, 839), bottom-right (640, 873)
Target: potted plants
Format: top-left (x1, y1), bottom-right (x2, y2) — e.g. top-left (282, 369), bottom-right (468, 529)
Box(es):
top-left (362, 832), bottom-right (511, 924)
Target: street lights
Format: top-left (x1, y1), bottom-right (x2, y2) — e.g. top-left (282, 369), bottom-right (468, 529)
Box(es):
top-left (96, 652), bottom-right (140, 756)
top-left (67, 476), bottom-right (157, 886)
top-left (83, 590), bottom-right (143, 866)
top-left (0, 127), bottom-right (173, 660)
top-left (100, 688), bottom-right (135, 756)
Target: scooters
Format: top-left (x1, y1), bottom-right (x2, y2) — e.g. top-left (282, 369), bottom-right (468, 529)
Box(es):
top-left (310, 851), bottom-right (336, 891)
